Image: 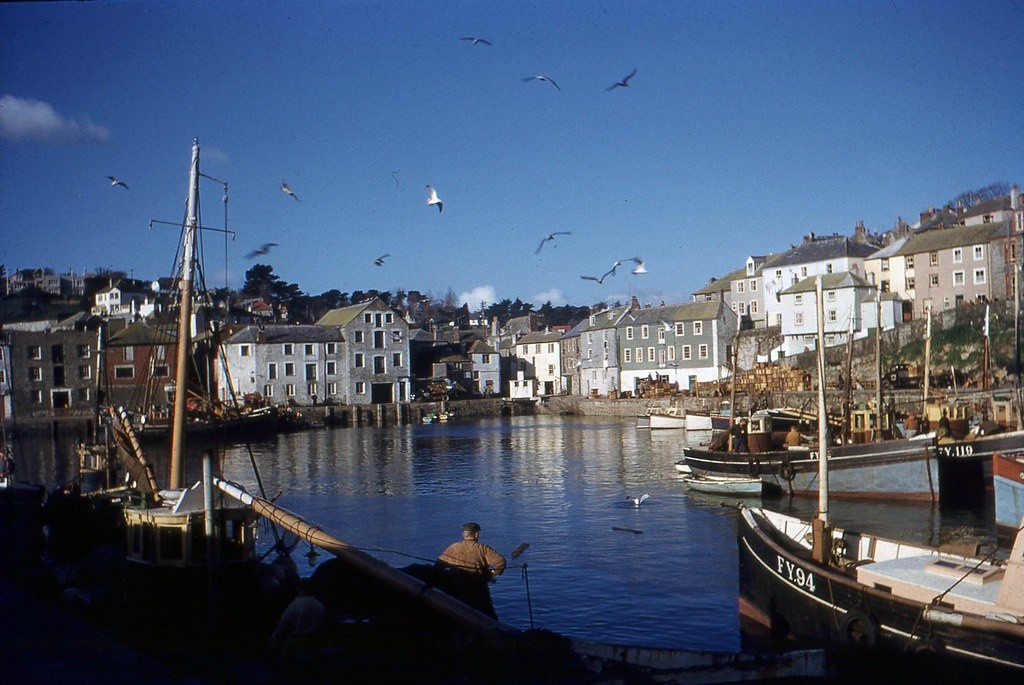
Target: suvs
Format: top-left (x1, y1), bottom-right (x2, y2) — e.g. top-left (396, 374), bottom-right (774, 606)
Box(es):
top-left (410, 385), bottom-right (449, 403)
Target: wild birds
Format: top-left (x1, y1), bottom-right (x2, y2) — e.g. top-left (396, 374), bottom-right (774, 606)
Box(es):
top-left (426, 184), bottom-right (442, 213)
top-left (570, 354), bottom-right (598, 367)
top-left (244, 242), bottom-right (278, 260)
top-left (628, 313), bottom-right (644, 321)
top-left (662, 320), bottom-right (675, 331)
top-left (581, 268), bottom-right (615, 283)
top-left (375, 254), bottom-right (392, 266)
top-left (522, 75), bottom-right (560, 91)
top-left (280, 178), bottom-right (299, 201)
top-left (667, 358), bottom-right (682, 368)
top-left (106, 175), bottom-right (128, 189)
top-left (461, 37), bottom-right (491, 46)
top-left (535, 232), bottom-right (571, 255)
top-left (606, 69), bottom-right (638, 92)
top-left (626, 494), bottom-right (651, 504)
top-left (614, 257), bottom-right (648, 275)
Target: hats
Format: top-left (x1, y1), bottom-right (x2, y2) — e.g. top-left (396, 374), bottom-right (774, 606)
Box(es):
top-left (462, 522), bottom-right (482, 532)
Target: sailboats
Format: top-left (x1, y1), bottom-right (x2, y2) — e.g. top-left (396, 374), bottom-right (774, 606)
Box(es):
top-left (0, 138), bottom-right (302, 664)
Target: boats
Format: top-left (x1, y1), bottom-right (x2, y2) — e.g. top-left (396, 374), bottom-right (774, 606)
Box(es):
top-left (132, 403), bottom-right (281, 443)
top-left (575, 637), bottom-right (832, 685)
top-left (636, 271), bottom-right (1024, 685)
top-left (422, 412), bottom-right (454, 422)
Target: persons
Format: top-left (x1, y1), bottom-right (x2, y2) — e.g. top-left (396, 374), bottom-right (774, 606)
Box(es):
top-left (266, 582), bottom-right (330, 663)
top-left (904, 412), bottom-right (918, 430)
top-left (732, 418), bottom-right (749, 452)
top-left (434, 522), bottom-right (506, 622)
top-left (786, 425), bottom-right (800, 446)
top-left (959, 292), bottom-right (1024, 306)
top-left (43, 481), bottom-right (86, 565)
